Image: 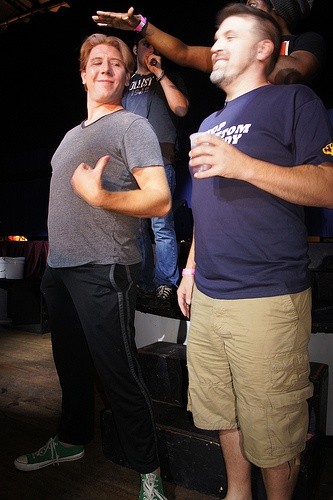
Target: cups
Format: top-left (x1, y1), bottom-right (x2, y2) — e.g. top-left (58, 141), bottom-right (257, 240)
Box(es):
top-left (190, 135), bottom-right (213, 172)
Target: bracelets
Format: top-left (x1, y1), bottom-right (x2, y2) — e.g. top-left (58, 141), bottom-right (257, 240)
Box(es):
top-left (181, 268), bottom-right (197, 275)
top-left (133, 14), bottom-right (148, 32)
top-left (154, 70), bottom-right (167, 83)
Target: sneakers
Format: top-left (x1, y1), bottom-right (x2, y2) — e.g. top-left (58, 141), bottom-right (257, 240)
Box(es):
top-left (13, 437), bottom-right (86, 472)
top-left (136, 468), bottom-right (168, 500)
top-left (135, 283), bottom-right (177, 309)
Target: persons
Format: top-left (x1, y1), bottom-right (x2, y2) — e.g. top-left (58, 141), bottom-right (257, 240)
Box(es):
top-left (119, 34), bottom-right (191, 310)
top-left (177, 4), bottom-right (333, 500)
top-left (12, 32), bottom-right (173, 500)
top-left (91, 0), bottom-right (332, 112)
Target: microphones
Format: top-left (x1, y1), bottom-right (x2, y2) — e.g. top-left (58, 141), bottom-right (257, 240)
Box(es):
top-left (151, 59), bottom-right (157, 66)
